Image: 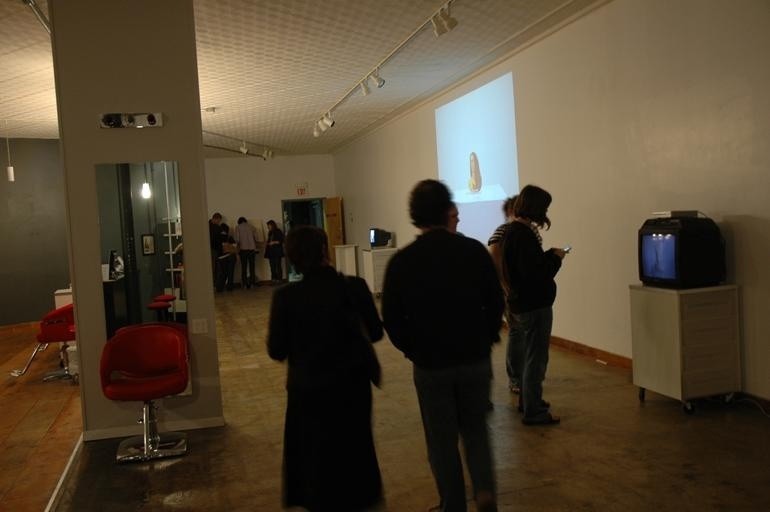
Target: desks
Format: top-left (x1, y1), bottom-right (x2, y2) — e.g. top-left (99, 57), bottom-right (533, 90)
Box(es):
top-left (333, 245), bottom-right (357, 277)
top-left (361, 248), bottom-right (403, 294)
top-left (102, 276), bottom-right (126, 337)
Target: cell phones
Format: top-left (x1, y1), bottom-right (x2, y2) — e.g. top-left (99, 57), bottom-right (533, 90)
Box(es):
top-left (563, 246), bottom-right (571, 253)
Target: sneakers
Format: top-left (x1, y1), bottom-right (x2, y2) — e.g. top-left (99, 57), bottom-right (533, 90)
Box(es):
top-left (509, 383), bottom-right (521, 394)
top-left (427, 502), bottom-right (468, 512)
top-left (521, 414), bottom-right (561, 425)
top-left (473, 489), bottom-right (499, 512)
top-left (517, 400), bottom-right (550, 411)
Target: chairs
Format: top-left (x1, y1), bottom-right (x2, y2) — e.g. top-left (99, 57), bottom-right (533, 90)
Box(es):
top-left (100, 324), bottom-right (187, 463)
top-left (7, 303), bottom-right (77, 385)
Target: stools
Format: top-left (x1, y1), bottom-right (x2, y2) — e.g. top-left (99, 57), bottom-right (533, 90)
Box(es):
top-left (153, 295), bottom-right (176, 318)
top-left (145, 302), bottom-right (172, 322)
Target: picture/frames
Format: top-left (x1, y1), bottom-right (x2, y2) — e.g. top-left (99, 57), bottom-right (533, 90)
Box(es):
top-left (141, 233), bottom-right (155, 256)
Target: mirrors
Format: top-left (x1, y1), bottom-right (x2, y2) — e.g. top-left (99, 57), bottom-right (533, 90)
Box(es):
top-left (96, 162), bottom-right (189, 342)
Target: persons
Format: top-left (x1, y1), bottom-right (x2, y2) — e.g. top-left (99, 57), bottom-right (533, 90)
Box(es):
top-left (208, 213), bottom-right (222, 289)
top-left (382, 179), bottom-right (504, 512)
top-left (487, 195), bottom-right (541, 393)
top-left (500, 184), bottom-right (566, 425)
top-left (235, 216), bottom-right (259, 289)
top-left (221, 224), bottom-right (237, 291)
top-left (264, 220), bottom-right (286, 282)
top-left (266, 227), bottom-right (384, 512)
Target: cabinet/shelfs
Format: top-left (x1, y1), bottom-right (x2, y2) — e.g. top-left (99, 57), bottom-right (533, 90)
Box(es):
top-left (160, 215), bottom-right (186, 321)
top-left (626, 283), bottom-right (740, 412)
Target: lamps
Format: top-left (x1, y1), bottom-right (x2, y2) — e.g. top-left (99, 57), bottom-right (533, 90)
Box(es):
top-left (431, 16), bottom-right (450, 39)
top-left (323, 113), bottom-right (335, 128)
top-left (142, 163), bottom-right (151, 199)
top-left (313, 121), bottom-right (319, 138)
top-left (239, 140), bottom-right (249, 154)
top-left (320, 119), bottom-right (329, 132)
top-left (4, 118), bottom-right (16, 182)
top-left (362, 78), bottom-right (372, 96)
top-left (369, 66), bottom-right (384, 88)
top-left (440, 3), bottom-right (458, 31)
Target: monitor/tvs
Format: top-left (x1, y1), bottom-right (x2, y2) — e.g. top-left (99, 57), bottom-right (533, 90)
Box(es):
top-left (638, 216), bottom-right (728, 291)
top-left (369, 228), bottom-right (392, 249)
